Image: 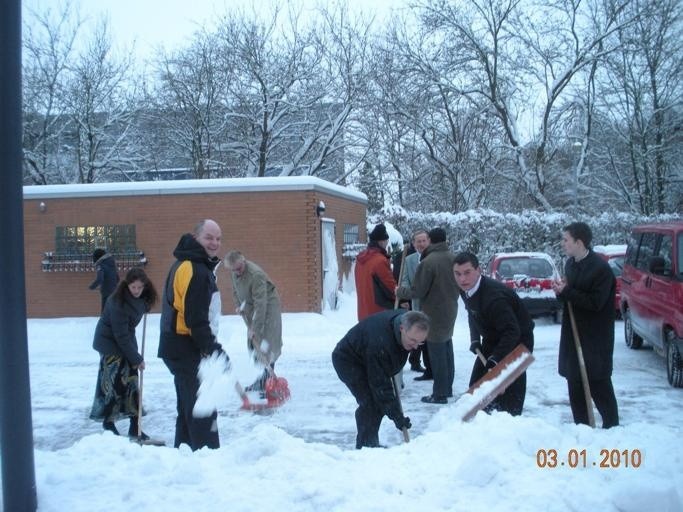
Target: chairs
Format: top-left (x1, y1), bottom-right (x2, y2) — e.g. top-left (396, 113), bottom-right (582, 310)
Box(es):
top-left (500, 264), bottom-right (511, 275)
top-left (529, 263), bottom-right (542, 274)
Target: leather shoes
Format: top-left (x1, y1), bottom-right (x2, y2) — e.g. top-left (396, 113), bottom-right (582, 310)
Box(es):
top-left (412, 363), bottom-right (432, 380)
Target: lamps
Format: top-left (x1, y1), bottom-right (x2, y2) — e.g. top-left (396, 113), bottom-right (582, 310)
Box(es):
top-left (317, 200), bottom-right (325, 217)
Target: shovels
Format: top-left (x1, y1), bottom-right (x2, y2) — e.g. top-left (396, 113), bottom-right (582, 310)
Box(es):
top-left (240, 311), bottom-right (290, 408)
top-left (459, 344), bottom-right (535, 421)
top-left (236, 383), bottom-right (274, 413)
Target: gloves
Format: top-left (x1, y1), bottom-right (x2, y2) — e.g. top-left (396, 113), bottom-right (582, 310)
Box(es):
top-left (486, 356), bottom-right (497, 368)
top-left (394, 414), bottom-right (411, 430)
top-left (470, 340), bottom-right (481, 354)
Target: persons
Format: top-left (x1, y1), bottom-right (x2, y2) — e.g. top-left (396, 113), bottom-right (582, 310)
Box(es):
top-left (332, 309), bottom-right (430, 449)
top-left (91, 268), bottom-right (158, 441)
top-left (396, 228), bottom-right (460, 404)
top-left (551, 222), bottom-right (619, 429)
top-left (88, 249), bottom-right (120, 315)
top-left (224, 251), bottom-right (281, 399)
top-left (399, 230), bottom-right (431, 372)
top-left (157, 218), bottom-right (230, 451)
top-left (355, 224), bottom-right (395, 324)
top-left (453, 252), bottom-right (535, 416)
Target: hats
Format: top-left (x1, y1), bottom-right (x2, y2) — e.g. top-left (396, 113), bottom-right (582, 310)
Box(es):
top-left (369, 224), bottom-right (388, 240)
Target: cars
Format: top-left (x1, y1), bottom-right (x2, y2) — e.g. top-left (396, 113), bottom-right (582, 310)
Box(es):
top-left (484, 253), bottom-right (568, 321)
top-left (594, 245), bottom-right (628, 316)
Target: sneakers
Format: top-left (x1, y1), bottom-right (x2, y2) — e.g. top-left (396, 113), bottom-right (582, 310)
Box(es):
top-left (421, 393), bottom-right (452, 403)
top-left (103, 420), bottom-right (149, 440)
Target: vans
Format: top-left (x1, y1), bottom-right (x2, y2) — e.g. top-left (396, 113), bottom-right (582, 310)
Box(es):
top-left (620, 225), bottom-right (683, 384)
